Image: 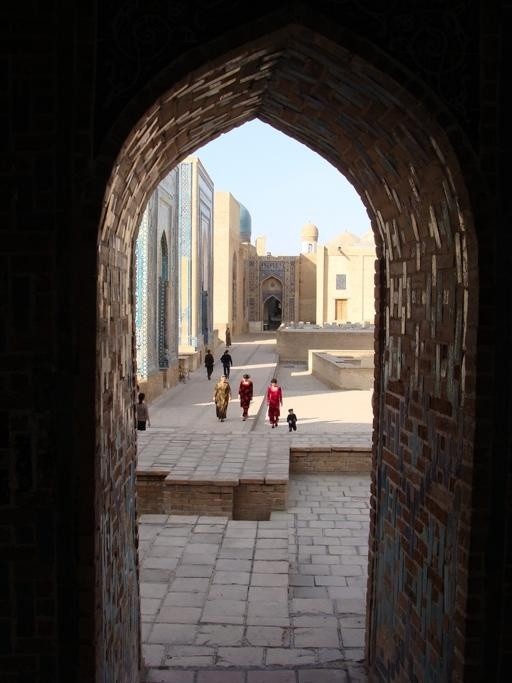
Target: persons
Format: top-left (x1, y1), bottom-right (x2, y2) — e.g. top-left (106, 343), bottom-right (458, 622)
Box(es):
top-left (136, 385), bottom-right (144, 404)
top-left (266, 379), bottom-right (283, 428)
top-left (287, 409), bottom-right (297, 432)
top-left (213, 375), bottom-right (232, 422)
top-left (226, 328), bottom-right (231, 347)
top-left (137, 393), bottom-right (150, 430)
top-left (221, 350), bottom-right (232, 379)
top-left (205, 350), bottom-right (215, 380)
top-left (238, 374), bottom-right (253, 421)
